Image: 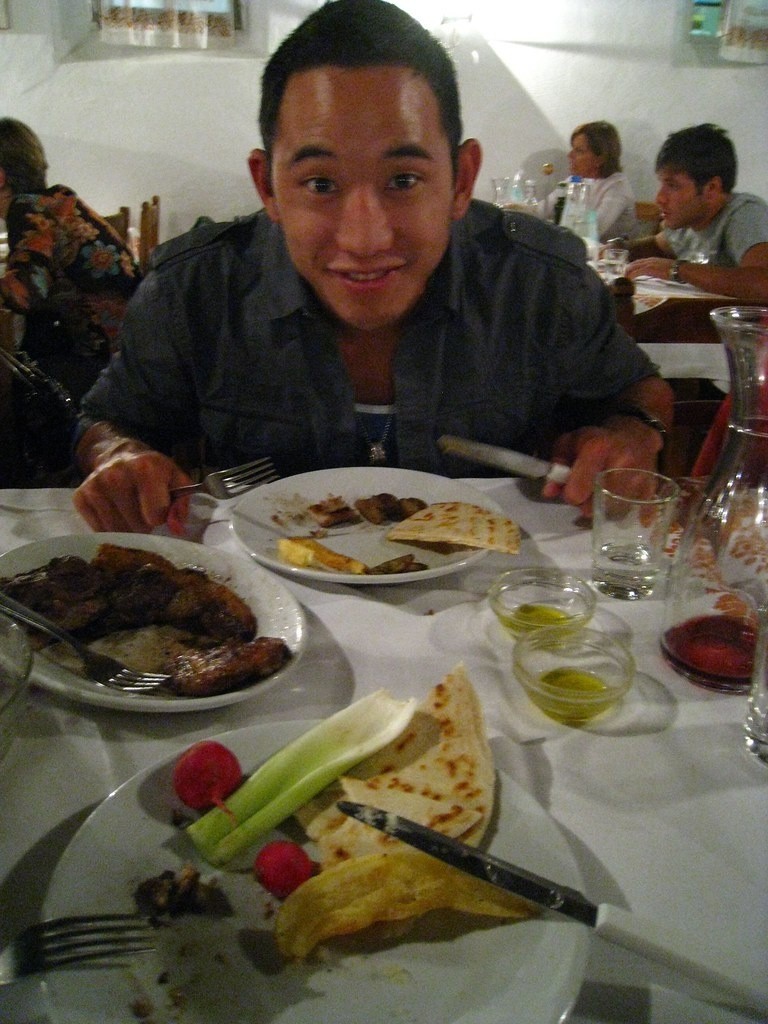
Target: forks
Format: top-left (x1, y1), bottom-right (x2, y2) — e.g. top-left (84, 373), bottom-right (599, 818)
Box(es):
top-left (0, 912), bottom-right (155, 985)
top-left (0, 587), bottom-right (171, 694)
top-left (168, 458), bottom-right (280, 503)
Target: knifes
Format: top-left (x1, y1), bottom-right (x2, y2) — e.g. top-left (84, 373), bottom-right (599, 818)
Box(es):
top-left (438, 435), bottom-right (570, 488)
top-left (338, 801), bottom-right (768, 1015)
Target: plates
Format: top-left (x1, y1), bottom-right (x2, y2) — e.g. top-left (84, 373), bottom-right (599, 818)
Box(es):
top-left (229, 465), bottom-right (504, 586)
top-left (39, 721), bottom-right (590, 1024)
top-left (0, 533), bottom-right (305, 713)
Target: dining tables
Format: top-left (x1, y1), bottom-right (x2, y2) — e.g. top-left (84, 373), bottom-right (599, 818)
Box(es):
top-left (0, 466), bottom-right (768, 1024)
top-left (584, 238), bottom-right (736, 343)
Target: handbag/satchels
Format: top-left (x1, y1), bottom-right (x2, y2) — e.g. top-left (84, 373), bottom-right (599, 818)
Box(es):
top-left (0, 348), bottom-right (79, 468)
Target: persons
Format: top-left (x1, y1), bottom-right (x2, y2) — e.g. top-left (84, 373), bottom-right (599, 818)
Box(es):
top-left (69, 1), bottom-right (672, 537)
top-left (599, 123), bottom-right (768, 299)
top-left (503, 121), bottom-right (637, 245)
top-left (0, 117), bottom-right (144, 479)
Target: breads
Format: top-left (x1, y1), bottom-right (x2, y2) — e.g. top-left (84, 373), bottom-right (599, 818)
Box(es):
top-left (278, 537), bottom-right (368, 574)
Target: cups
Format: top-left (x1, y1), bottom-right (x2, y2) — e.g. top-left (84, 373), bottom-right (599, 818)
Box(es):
top-left (0, 613), bottom-right (34, 772)
top-left (605, 249), bottom-right (628, 281)
top-left (739, 614), bottom-right (768, 762)
top-left (592, 468), bottom-right (678, 600)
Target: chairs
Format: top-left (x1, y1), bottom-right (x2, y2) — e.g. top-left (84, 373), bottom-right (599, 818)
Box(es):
top-left (628, 201), bottom-right (662, 231)
top-left (137, 194), bottom-right (163, 262)
top-left (101, 202), bottom-right (131, 242)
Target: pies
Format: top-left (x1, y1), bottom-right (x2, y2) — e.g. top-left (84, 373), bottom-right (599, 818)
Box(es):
top-left (295, 672), bottom-right (494, 877)
top-left (386, 501), bottom-right (521, 555)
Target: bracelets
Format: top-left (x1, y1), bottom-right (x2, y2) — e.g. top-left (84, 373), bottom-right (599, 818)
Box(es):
top-left (607, 406), bottom-right (671, 447)
top-left (670, 260), bottom-right (688, 284)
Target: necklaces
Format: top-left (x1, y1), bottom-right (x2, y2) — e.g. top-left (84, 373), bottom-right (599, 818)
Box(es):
top-left (354, 402), bottom-right (393, 463)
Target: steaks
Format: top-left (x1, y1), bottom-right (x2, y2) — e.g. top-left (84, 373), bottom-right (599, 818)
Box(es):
top-left (161, 634), bottom-right (289, 698)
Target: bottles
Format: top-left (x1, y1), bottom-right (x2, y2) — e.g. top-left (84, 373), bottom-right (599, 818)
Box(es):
top-left (656, 304), bottom-right (768, 695)
top-left (490, 175), bottom-right (600, 272)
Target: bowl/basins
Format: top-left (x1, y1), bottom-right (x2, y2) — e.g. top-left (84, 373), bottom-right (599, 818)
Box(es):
top-left (509, 629), bottom-right (635, 726)
top-left (486, 566), bottom-right (597, 641)
